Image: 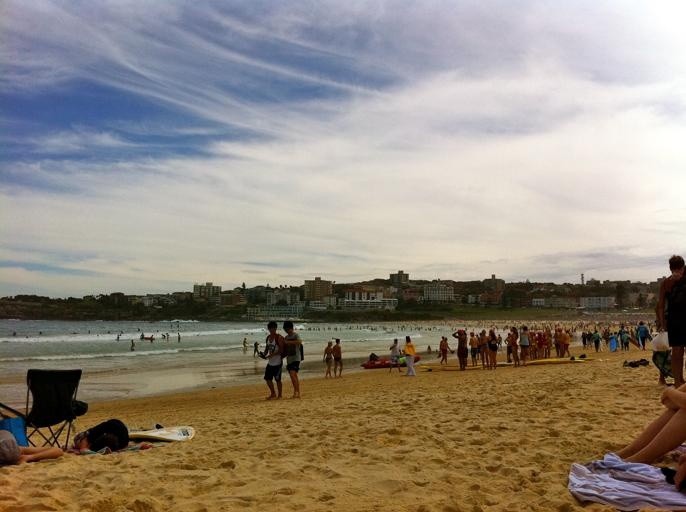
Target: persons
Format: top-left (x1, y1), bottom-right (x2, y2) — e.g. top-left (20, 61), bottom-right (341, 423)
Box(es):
top-left (663, 450), bottom-right (686, 495)
top-left (667, 266), bottom-right (685, 389)
top-left (0, 426), bottom-right (62, 469)
top-left (12, 323), bottom-right (182, 351)
top-left (609, 382), bottom-right (686, 465)
top-left (657, 255), bottom-right (683, 388)
top-left (70, 419), bottom-right (130, 457)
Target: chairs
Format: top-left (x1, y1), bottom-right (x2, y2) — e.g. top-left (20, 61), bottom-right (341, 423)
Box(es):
top-left (0, 366), bottom-right (89, 452)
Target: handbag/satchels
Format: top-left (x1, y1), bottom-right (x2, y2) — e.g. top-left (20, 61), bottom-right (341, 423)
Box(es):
top-left (1, 416), bottom-right (30, 448)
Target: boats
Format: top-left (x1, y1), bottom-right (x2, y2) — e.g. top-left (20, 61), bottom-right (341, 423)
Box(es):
top-left (361, 353), bottom-right (420, 370)
